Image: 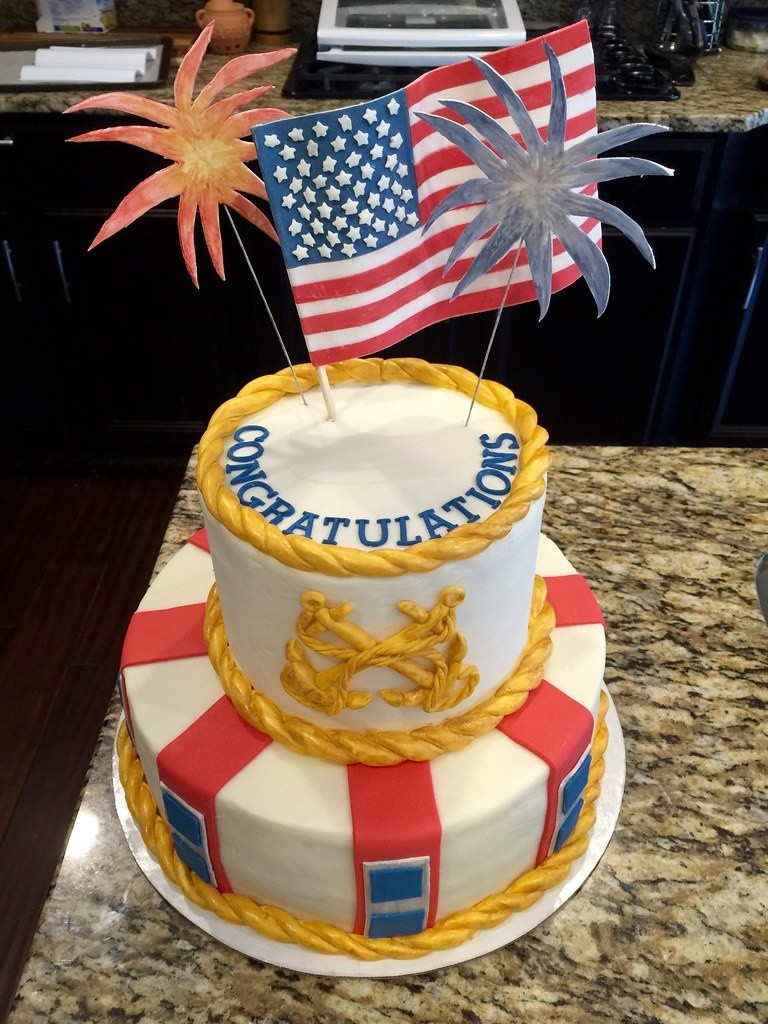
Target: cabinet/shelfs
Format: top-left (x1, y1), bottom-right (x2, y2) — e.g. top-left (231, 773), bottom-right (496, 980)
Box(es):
top-left (0, 112), bottom-right (768, 479)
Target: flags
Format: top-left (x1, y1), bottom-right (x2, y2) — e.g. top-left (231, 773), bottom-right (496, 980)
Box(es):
top-left (251, 18), bottom-right (601, 366)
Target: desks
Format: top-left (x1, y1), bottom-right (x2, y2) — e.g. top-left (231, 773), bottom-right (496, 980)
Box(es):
top-left (5, 441), bottom-right (768, 1024)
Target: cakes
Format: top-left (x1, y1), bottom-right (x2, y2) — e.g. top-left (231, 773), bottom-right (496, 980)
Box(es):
top-left (62, 17), bottom-right (675, 961)
